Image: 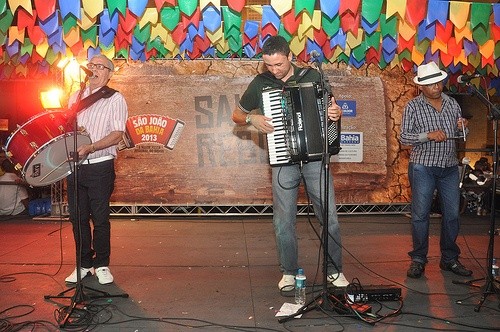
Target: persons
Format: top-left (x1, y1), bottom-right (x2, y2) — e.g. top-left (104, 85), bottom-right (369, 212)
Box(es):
top-left (63, 54), bottom-right (129, 284)
top-left (399, 61), bottom-right (473, 277)
top-left (0, 158), bottom-right (29, 216)
top-left (232, 34), bottom-right (350, 291)
top-left (462, 156), bottom-right (494, 209)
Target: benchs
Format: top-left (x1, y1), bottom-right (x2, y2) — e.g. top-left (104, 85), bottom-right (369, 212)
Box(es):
top-left (459, 164), bottom-right (492, 216)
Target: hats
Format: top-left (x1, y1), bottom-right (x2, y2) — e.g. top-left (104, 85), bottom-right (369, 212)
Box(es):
top-left (413, 61), bottom-right (447, 85)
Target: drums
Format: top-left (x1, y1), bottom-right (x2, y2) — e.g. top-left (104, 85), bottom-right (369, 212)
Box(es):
top-left (2, 108), bottom-right (93, 188)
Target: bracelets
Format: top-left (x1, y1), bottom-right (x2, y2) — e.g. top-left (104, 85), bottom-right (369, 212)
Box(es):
top-left (91, 144), bottom-right (97, 154)
top-left (246, 113), bottom-right (250, 126)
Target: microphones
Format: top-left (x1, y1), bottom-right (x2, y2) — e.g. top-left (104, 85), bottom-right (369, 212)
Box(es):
top-left (311, 50), bottom-right (321, 68)
top-left (457, 74), bottom-right (480, 84)
top-left (80, 65), bottom-right (95, 79)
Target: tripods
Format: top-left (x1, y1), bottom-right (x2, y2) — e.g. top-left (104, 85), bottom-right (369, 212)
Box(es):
top-left (44, 79), bottom-right (130, 329)
top-left (278, 67), bottom-right (375, 325)
top-left (453, 80), bottom-right (500, 312)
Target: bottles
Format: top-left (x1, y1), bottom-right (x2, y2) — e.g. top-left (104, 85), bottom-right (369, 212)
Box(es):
top-left (295, 269), bottom-right (306, 305)
top-left (492, 259), bottom-right (497, 279)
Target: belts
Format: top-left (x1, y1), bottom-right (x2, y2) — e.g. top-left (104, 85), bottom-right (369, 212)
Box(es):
top-left (81, 154), bottom-right (114, 164)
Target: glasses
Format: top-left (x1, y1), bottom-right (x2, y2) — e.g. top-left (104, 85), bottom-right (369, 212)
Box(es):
top-left (87, 63), bottom-right (111, 70)
top-left (419, 81), bottom-right (442, 88)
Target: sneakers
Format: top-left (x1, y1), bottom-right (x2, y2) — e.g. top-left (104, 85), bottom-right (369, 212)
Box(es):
top-left (64, 266), bottom-right (95, 283)
top-left (439, 256), bottom-right (472, 276)
top-left (278, 275), bottom-right (296, 291)
top-left (327, 273), bottom-right (350, 288)
top-left (95, 267), bottom-right (114, 285)
top-left (407, 261), bottom-right (425, 278)
